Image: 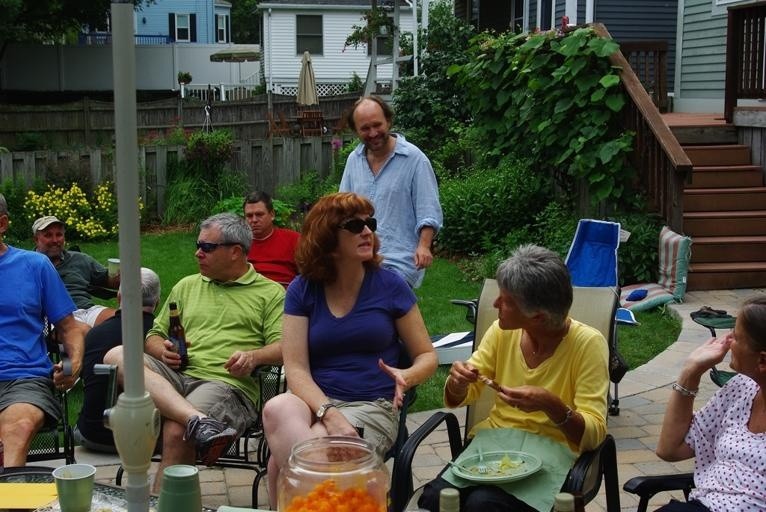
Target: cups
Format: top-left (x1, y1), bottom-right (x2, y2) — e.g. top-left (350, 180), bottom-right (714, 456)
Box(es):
top-left (50, 462), bottom-right (95, 511)
top-left (154, 464), bottom-right (203, 511)
top-left (106, 256), bottom-right (119, 276)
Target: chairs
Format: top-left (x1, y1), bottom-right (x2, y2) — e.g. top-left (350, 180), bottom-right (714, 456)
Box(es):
top-left (264, 108), bottom-right (351, 137)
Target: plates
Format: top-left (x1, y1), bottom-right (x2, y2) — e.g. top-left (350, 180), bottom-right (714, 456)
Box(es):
top-left (451, 449), bottom-right (542, 485)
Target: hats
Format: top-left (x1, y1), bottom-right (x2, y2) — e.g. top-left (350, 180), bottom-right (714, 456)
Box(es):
top-left (32, 216), bottom-right (65, 237)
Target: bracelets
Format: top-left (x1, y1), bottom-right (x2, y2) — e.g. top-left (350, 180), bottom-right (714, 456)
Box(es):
top-left (670, 382), bottom-right (701, 400)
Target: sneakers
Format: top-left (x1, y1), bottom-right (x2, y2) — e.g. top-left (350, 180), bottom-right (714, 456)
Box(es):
top-left (183, 414), bottom-right (238, 466)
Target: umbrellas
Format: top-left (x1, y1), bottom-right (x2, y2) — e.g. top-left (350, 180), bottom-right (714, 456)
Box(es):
top-left (294, 48), bottom-right (321, 114)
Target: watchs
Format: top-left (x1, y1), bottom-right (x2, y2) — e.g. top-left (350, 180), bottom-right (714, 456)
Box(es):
top-left (552, 406), bottom-right (574, 426)
top-left (313, 402), bottom-right (336, 422)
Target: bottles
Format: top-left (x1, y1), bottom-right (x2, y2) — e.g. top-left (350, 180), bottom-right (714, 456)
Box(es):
top-left (273, 435), bottom-right (392, 512)
top-left (158, 300), bottom-right (191, 373)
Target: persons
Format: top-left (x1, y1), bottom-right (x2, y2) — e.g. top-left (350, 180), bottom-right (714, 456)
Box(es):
top-left (101, 210), bottom-right (287, 498)
top-left (651, 294), bottom-right (766, 511)
top-left (0, 192), bottom-right (86, 470)
top-left (416, 241), bottom-right (611, 512)
top-left (30, 214), bottom-right (120, 345)
top-left (259, 192), bottom-right (439, 512)
top-left (70, 265), bottom-right (164, 455)
top-left (337, 93), bottom-right (446, 294)
top-left (240, 189), bottom-right (301, 293)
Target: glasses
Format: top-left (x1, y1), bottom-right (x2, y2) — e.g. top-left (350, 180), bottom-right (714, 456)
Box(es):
top-left (338, 218), bottom-right (376, 233)
top-left (196, 240), bottom-right (243, 252)
